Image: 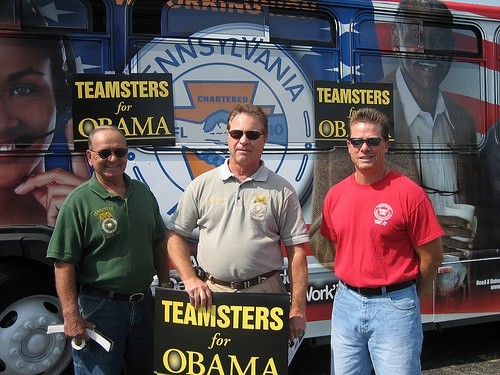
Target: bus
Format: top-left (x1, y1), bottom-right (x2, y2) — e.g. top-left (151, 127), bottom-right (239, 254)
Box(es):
top-left (0, 0), bottom-right (500, 375)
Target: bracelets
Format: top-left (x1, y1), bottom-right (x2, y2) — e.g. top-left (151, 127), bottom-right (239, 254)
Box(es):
top-left (160, 282), bottom-right (172, 288)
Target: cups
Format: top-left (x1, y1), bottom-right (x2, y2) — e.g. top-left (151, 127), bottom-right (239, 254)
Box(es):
top-left (444, 204), bottom-right (475, 241)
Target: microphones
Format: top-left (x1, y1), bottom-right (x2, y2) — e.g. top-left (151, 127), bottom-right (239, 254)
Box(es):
top-left (15, 129), bottom-right (56, 149)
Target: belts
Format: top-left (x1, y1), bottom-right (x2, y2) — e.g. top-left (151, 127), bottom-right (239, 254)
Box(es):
top-left (83, 286), bottom-right (152, 304)
top-left (340, 279), bottom-right (415, 297)
top-left (436, 282), bottom-right (466, 312)
top-left (204, 269), bottom-right (283, 290)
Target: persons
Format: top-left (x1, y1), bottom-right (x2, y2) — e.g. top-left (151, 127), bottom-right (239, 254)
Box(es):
top-left (377, 0), bottom-right (483, 296)
top-left (320, 107), bottom-right (443, 375)
top-left (53, 125), bottom-right (171, 375)
top-left (165, 105), bottom-right (308, 345)
top-left (1, 1), bottom-right (93, 229)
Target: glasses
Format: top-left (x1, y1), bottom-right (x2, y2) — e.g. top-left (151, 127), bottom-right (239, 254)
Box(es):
top-left (416, 136), bottom-right (460, 197)
top-left (228, 130), bottom-right (263, 140)
top-left (348, 137), bottom-right (386, 147)
top-left (91, 148), bottom-right (128, 160)
